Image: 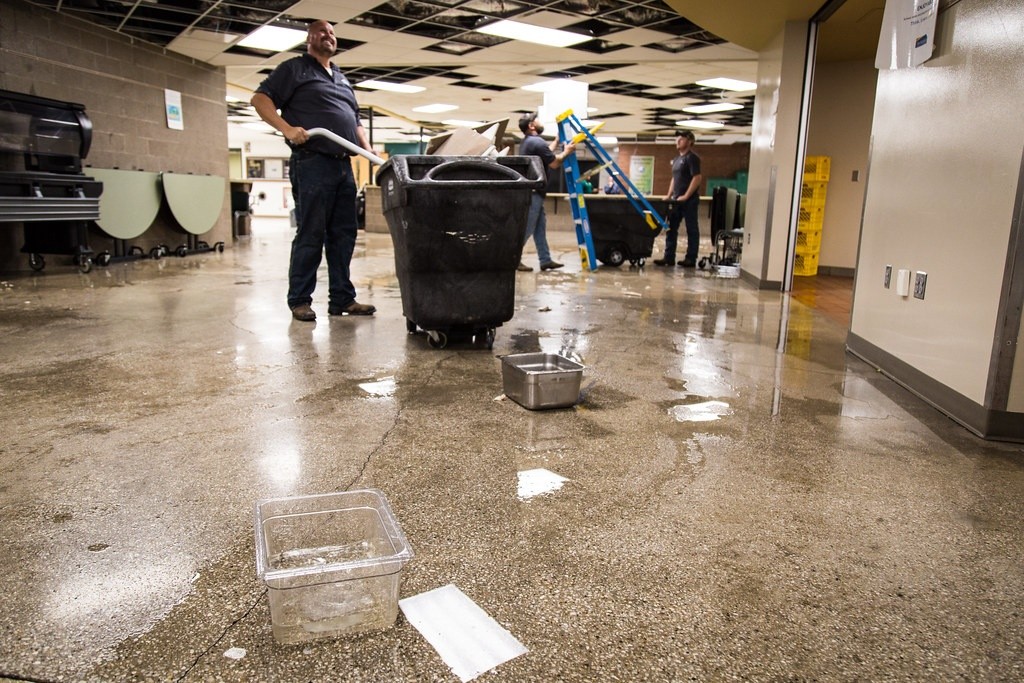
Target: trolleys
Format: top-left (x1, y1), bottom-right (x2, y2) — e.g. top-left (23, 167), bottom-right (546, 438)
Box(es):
top-left (565, 193), bottom-right (682, 268)
top-left (375, 153), bottom-right (548, 349)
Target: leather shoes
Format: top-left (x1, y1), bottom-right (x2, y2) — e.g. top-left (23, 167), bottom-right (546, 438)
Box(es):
top-left (328, 301), bottom-right (376, 315)
top-left (291, 303), bottom-right (316, 321)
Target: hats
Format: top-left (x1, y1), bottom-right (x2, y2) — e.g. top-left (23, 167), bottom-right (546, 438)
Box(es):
top-left (675, 130), bottom-right (695, 142)
top-left (519, 111), bottom-right (538, 131)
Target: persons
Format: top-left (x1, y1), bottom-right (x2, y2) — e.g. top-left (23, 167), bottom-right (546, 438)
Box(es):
top-left (250, 21), bottom-right (378, 321)
top-left (604, 175), bottom-right (620, 194)
top-left (517, 112), bottom-right (576, 271)
top-left (582, 171), bottom-right (593, 193)
top-left (653, 130), bottom-right (702, 267)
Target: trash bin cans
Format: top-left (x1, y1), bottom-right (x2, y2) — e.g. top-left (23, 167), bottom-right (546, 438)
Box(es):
top-left (583, 198), bottom-right (667, 267)
top-left (374, 153), bottom-right (549, 349)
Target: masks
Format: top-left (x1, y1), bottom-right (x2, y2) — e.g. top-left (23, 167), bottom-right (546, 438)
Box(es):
top-left (532, 122), bottom-right (544, 135)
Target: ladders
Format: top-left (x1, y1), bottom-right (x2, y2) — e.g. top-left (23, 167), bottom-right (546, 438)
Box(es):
top-left (555, 109), bottom-right (672, 273)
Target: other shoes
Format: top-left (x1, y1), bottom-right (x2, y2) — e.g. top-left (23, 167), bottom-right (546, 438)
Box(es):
top-left (678, 258), bottom-right (696, 267)
top-left (517, 263), bottom-right (533, 271)
top-left (653, 259), bottom-right (675, 266)
top-left (541, 261), bottom-right (563, 270)
